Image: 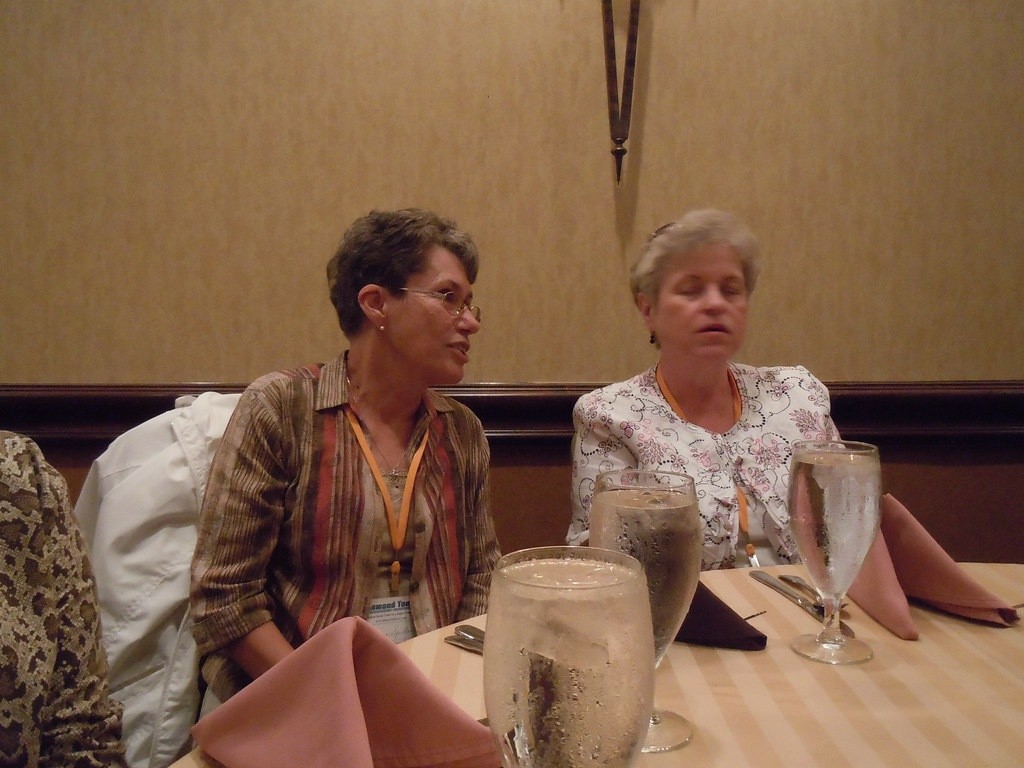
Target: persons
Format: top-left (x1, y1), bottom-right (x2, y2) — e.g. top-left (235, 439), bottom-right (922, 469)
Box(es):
top-left (0, 431), bottom-right (129, 768)
top-left (565, 209), bottom-right (845, 571)
top-left (191, 209), bottom-right (501, 750)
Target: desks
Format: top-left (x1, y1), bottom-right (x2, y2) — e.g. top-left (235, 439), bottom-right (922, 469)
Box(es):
top-left (166, 562), bottom-right (1024, 768)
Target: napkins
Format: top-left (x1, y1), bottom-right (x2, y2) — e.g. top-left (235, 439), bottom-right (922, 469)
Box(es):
top-left (191, 614), bottom-right (510, 768)
top-left (796, 467), bottom-right (1024, 643)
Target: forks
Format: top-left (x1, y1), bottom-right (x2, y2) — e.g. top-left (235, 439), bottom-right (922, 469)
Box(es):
top-left (778, 575), bottom-right (849, 609)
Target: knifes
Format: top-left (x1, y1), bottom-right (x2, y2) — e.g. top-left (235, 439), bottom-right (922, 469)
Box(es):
top-left (749, 570), bottom-right (856, 638)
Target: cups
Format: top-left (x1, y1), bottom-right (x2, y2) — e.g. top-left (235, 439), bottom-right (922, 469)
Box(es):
top-left (482, 546), bottom-right (657, 768)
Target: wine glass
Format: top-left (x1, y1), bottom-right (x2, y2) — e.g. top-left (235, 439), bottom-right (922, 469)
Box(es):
top-left (789, 439), bottom-right (881, 666)
top-left (588, 469), bottom-right (702, 754)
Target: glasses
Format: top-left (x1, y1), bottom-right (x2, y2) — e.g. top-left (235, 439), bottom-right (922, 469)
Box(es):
top-left (395, 287), bottom-right (481, 322)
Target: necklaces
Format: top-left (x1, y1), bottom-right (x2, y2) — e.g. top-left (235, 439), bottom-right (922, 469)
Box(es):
top-left (347, 376), bottom-right (408, 482)
